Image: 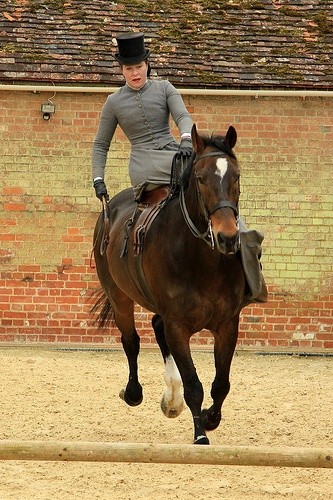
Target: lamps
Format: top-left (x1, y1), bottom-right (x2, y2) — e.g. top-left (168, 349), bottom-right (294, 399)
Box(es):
top-left (40, 104), bottom-right (54, 121)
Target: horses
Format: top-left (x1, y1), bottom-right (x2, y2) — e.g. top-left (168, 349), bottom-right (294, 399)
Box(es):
top-left (93, 124), bottom-right (268, 444)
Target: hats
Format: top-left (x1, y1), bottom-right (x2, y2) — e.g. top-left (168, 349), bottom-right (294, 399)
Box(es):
top-left (112, 32), bottom-right (150, 66)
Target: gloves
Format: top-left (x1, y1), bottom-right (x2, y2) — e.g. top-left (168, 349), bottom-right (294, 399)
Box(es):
top-left (177, 137), bottom-right (193, 161)
top-left (93, 179), bottom-right (109, 201)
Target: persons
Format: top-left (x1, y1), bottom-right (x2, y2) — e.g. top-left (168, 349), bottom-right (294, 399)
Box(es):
top-left (90, 33), bottom-right (268, 303)
